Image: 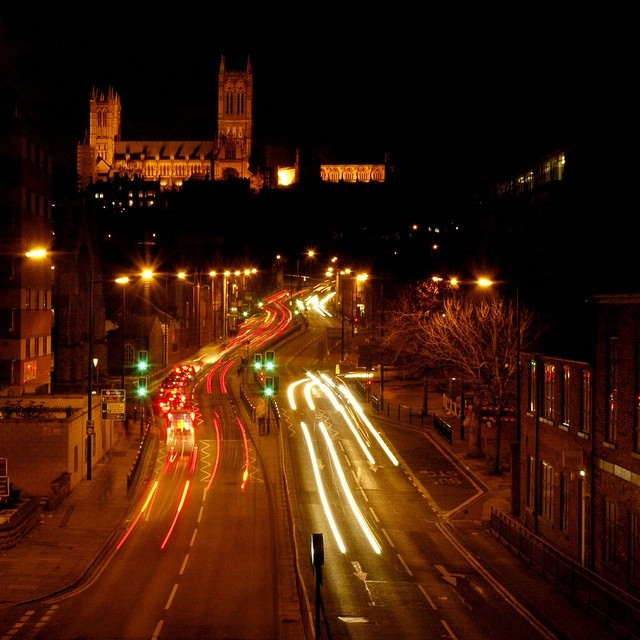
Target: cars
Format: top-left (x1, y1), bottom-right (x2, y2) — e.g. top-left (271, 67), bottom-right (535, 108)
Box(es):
top-left (152, 359), bottom-right (205, 445)
top-left (343, 366), bottom-right (374, 382)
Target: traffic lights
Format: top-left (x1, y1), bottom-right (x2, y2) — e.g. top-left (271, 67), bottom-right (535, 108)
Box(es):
top-left (139, 350), bottom-right (148, 373)
top-left (263, 377), bottom-right (273, 396)
top-left (309, 529), bottom-right (325, 640)
top-left (266, 351), bottom-right (275, 372)
top-left (137, 376), bottom-right (147, 398)
top-left (259, 371), bottom-right (264, 383)
top-left (254, 354), bottom-right (262, 372)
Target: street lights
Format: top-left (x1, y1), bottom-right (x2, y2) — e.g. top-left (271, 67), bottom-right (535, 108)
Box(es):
top-left (194, 266), bottom-right (216, 350)
top-left (272, 253), bottom-right (283, 292)
top-left (567, 469), bottom-right (587, 588)
top-left (219, 268), bottom-right (231, 339)
top-left (296, 248), bottom-right (315, 296)
top-left (323, 255), bottom-right (352, 362)
top-left (243, 264), bottom-right (258, 317)
top-left (236, 265), bottom-right (251, 323)
top-left (481, 279), bottom-right (521, 451)
top-left (227, 269), bottom-right (242, 334)
top-left (119, 263), bottom-right (158, 438)
top-left (449, 277), bottom-right (477, 442)
top-left (163, 266), bottom-right (186, 368)
top-left (84, 275), bottom-right (132, 482)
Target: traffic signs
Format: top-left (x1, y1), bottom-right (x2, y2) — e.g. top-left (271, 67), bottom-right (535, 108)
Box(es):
top-left (100, 389), bottom-right (127, 421)
